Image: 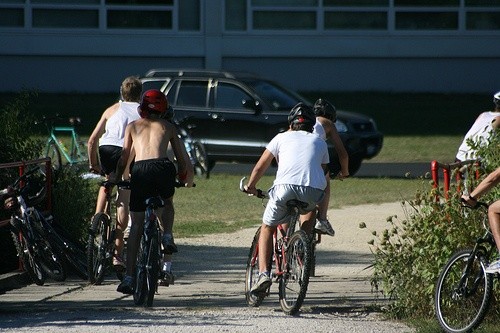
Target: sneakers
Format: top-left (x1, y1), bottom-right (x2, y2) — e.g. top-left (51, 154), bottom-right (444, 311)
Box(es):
top-left (91, 217), bottom-right (104, 247)
top-left (117, 280), bottom-right (131, 293)
top-left (251, 273), bottom-right (271, 292)
top-left (486, 260), bottom-right (500, 273)
top-left (315, 220), bottom-right (334, 236)
top-left (162, 237), bottom-right (178, 253)
top-left (113, 258), bottom-right (125, 269)
top-left (162, 270), bottom-right (174, 284)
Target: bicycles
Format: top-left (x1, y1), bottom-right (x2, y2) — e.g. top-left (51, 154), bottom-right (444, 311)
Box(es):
top-left (0, 165), bottom-right (89, 286)
top-left (171, 121), bottom-right (211, 181)
top-left (237, 176), bottom-right (311, 316)
top-left (83, 166), bottom-right (125, 286)
top-left (295, 174), bottom-right (345, 276)
top-left (434, 198), bottom-right (500, 333)
top-left (119, 177), bottom-right (196, 306)
top-left (35, 115), bottom-right (89, 178)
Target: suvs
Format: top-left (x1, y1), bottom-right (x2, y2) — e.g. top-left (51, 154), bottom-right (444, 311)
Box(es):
top-left (131, 68), bottom-right (383, 178)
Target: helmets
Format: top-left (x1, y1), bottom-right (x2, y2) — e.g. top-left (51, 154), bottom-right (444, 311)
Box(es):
top-left (493, 92), bottom-right (500, 103)
top-left (142, 90), bottom-right (167, 111)
top-left (314, 99), bottom-right (337, 124)
top-left (288, 102), bottom-right (316, 126)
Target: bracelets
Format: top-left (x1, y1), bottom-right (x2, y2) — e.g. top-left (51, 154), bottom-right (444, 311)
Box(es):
top-left (469, 193), bottom-right (477, 201)
top-left (178, 170), bottom-right (188, 180)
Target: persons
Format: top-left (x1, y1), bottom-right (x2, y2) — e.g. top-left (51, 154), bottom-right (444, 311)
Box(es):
top-left (312, 98), bottom-right (349, 236)
top-left (461, 165), bottom-right (500, 274)
top-left (87, 76), bottom-right (156, 272)
top-left (455, 90), bottom-right (500, 176)
top-left (243, 102), bottom-right (329, 294)
top-left (117, 91), bottom-right (195, 293)
